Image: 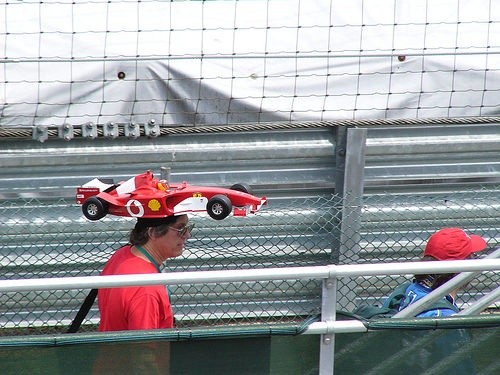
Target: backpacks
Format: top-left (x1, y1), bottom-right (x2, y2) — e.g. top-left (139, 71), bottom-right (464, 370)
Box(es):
top-left (352, 282), bottom-right (416, 319)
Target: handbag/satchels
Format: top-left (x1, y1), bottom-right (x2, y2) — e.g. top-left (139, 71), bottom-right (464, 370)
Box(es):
top-left (57, 343), bottom-right (95, 375)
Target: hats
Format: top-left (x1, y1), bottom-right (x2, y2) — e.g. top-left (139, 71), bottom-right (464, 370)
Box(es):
top-left (424, 227), bottom-right (488, 261)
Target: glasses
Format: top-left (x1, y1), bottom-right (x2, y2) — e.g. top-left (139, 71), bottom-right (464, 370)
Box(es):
top-left (169, 221), bottom-right (195, 238)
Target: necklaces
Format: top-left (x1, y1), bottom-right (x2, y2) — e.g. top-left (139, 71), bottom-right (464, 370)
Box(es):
top-left (136, 244), bottom-right (172, 304)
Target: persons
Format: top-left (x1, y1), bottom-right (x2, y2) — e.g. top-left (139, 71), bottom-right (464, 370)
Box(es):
top-left (383, 228), bottom-right (487, 375)
top-left (94, 212), bottom-right (195, 374)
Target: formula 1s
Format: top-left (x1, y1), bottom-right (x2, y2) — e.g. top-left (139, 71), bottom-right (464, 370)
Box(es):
top-left (76, 169), bottom-right (268, 221)
